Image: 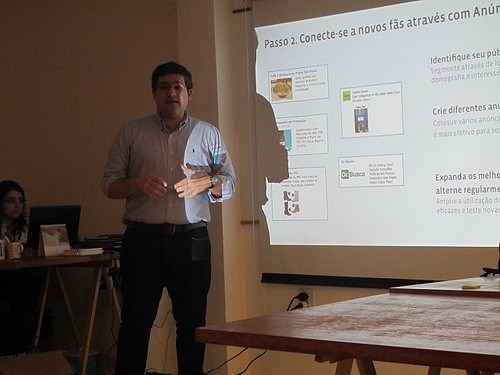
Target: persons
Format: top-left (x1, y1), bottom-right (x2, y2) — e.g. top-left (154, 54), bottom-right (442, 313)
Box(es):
top-left (101, 61), bottom-right (236, 375)
top-left (0, 180), bottom-right (56, 357)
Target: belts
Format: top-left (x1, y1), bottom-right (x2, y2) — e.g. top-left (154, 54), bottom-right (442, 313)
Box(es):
top-left (127, 221), bottom-right (207, 235)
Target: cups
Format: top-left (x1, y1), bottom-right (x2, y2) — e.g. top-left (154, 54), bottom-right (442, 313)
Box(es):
top-left (7, 241), bottom-right (24, 260)
top-left (0, 240), bottom-right (6, 260)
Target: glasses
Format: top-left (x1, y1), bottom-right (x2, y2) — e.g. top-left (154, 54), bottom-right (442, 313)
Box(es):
top-left (3, 198), bottom-right (27, 204)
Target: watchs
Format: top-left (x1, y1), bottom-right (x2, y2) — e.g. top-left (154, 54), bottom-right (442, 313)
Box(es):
top-left (209, 173), bottom-right (217, 189)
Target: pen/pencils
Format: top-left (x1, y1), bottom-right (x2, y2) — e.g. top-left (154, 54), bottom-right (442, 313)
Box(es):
top-left (3, 228), bottom-right (24, 242)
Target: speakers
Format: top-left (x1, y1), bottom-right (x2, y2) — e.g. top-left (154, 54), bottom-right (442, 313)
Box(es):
top-left (0, 350), bottom-right (80, 375)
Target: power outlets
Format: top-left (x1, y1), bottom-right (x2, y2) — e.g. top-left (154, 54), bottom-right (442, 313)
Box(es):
top-left (297, 288), bottom-right (314, 308)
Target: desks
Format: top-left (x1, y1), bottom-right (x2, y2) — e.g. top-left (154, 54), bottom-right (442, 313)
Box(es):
top-left (195, 277), bottom-right (500, 375)
top-left (0, 251), bottom-right (120, 375)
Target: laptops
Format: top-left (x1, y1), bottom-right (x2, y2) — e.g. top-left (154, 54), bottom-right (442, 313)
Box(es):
top-left (20, 205), bottom-right (81, 257)
